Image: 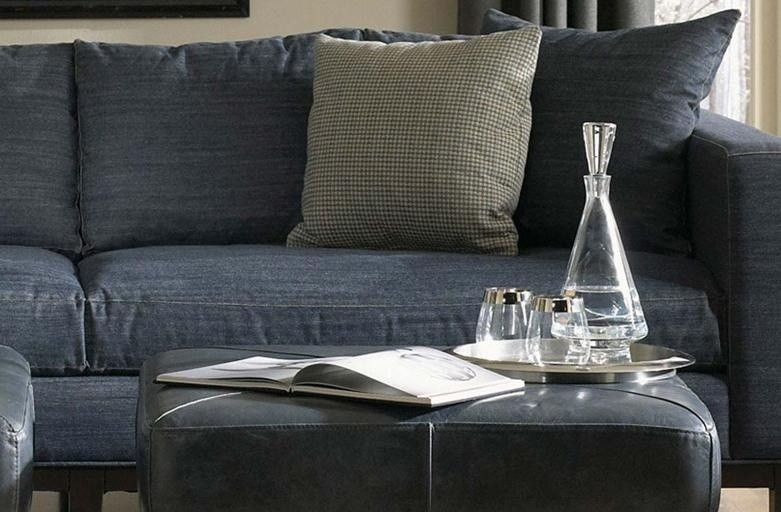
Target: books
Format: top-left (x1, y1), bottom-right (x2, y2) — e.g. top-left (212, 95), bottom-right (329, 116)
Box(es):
top-left (153, 346), bottom-right (527, 409)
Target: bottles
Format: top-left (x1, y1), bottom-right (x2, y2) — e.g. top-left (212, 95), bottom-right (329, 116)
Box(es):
top-left (551, 124), bottom-right (650, 360)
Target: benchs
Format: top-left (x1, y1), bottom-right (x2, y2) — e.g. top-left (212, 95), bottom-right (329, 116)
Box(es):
top-left (135, 343), bottom-right (721, 511)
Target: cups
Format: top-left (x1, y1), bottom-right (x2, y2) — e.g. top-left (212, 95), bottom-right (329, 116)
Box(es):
top-left (525, 293), bottom-right (591, 368)
top-left (475, 286), bottom-right (541, 364)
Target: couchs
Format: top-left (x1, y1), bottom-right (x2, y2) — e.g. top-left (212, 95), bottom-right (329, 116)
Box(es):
top-left (1, 28), bottom-right (781, 511)
top-left (0, 346), bottom-right (35, 511)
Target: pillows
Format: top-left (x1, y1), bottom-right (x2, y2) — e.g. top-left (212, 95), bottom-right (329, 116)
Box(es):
top-left (285, 24), bottom-right (542, 255)
top-left (480, 7), bottom-right (741, 257)
top-left (1, 42), bottom-right (83, 254)
top-left (74, 29), bottom-right (365, 259)
top-left (364, 27), bottom-right (480, 44)
